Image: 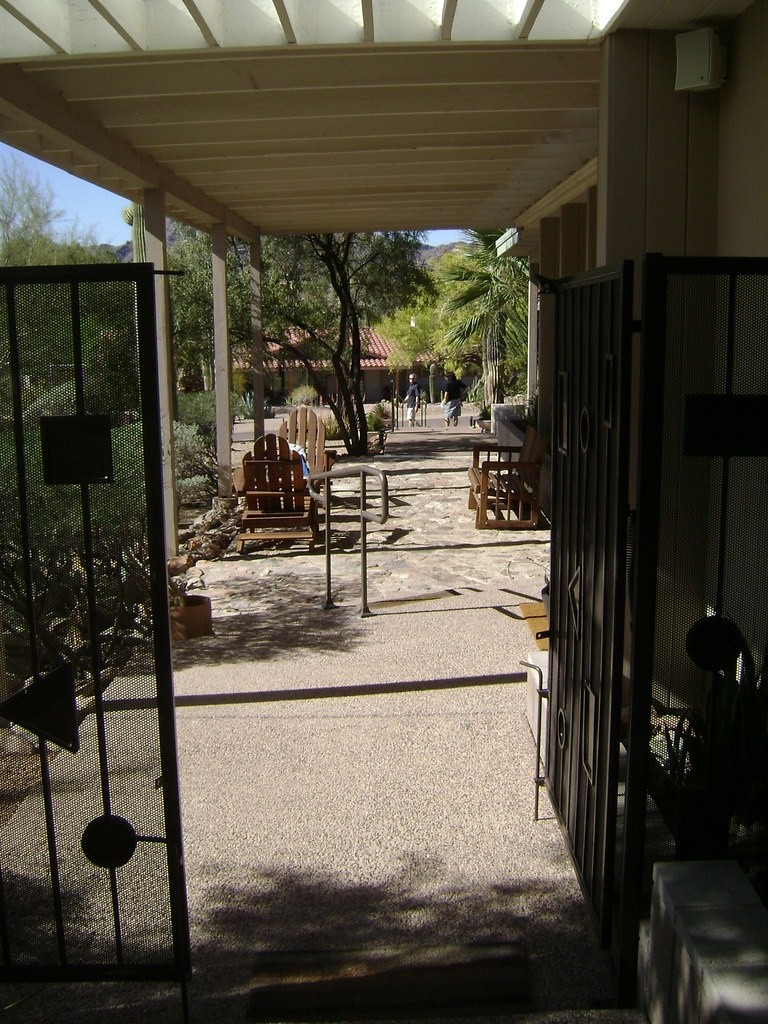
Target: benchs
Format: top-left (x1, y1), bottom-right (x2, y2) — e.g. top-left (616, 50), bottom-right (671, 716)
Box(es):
top-left (468, 425), bottom-right (549, 530)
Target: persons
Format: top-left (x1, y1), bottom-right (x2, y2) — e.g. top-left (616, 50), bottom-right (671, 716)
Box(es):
top-left (400, 373), bottom-right (420, 426)
top-left (441, 372), bottom-right (469, 427)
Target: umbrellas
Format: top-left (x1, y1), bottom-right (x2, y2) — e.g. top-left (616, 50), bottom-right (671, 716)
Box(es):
top-left (270, 334), bottom-right (388, 361)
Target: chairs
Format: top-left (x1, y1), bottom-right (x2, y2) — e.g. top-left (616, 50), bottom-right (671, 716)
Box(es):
top-left (280, 407), bottom-right (336, 466)
top-left (236, 434), bottom-right (322, 553)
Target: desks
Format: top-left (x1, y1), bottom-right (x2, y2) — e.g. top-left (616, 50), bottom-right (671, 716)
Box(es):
top-left (234, 469), bottom-right (323, 538)
